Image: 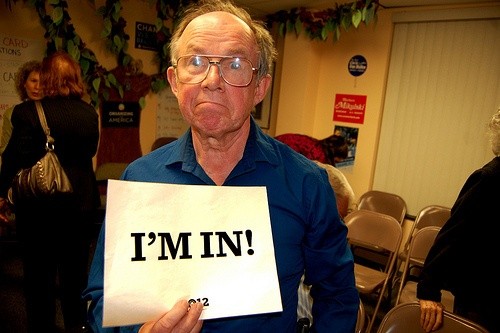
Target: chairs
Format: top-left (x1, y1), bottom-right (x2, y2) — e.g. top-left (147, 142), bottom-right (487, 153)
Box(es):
top-left (298, 190), bottom-right (487, 333)
top-left (96, 163), bottom-right (129, 210)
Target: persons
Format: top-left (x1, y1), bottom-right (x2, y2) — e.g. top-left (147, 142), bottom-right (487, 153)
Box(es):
top-left (0, 50), bottom-right (101, 333)
top-left (273, 134), bottom-right (348, 167)
top-left (416, 107), bottom-right (500, 333)
top-left (82, 0), bottom-right (360, 333)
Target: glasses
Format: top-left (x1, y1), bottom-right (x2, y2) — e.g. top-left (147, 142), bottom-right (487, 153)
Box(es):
top-left (172, 54), bottom-right (259, 87)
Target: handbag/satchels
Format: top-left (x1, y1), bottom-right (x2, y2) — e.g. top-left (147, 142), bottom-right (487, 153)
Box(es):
top-left (11, 151), bottom-right (74, 198)
